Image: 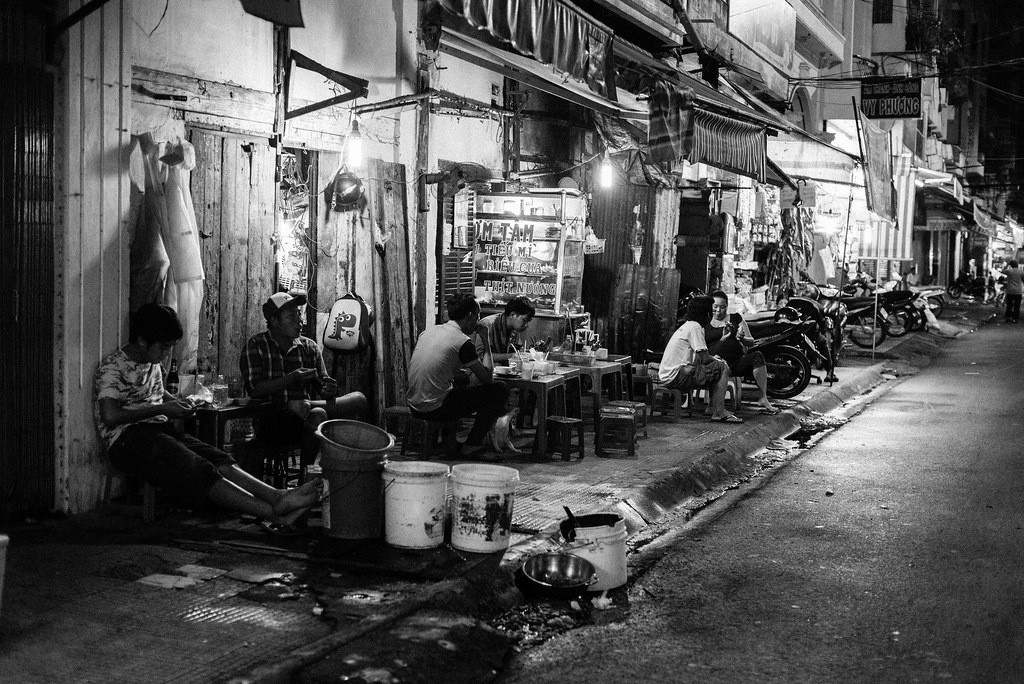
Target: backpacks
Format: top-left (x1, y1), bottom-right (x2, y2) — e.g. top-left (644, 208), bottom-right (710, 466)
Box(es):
top-left (322, 294), bottom-right (369, 351)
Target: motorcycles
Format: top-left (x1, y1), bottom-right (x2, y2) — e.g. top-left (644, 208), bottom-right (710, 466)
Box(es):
top-left (795, 272), bottom-right (895, 348)
top-left (690, 288), bottom-right (846, 400)
top-left (947, 271), bottom-right (1007, 308)
top-left (847, 273), bottom-right (945, 337)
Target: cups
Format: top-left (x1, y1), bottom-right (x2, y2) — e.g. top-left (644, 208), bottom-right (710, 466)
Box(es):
top-left (521, 361), bottom-right (534, 381)
top-left (597, 348), bottom-right (608, 359)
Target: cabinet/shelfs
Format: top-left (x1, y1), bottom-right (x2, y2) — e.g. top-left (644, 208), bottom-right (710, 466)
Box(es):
top-left (474, 187), bottom-right (587, 316)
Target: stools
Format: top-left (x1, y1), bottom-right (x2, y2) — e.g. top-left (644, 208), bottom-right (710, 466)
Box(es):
top-left (532, 415), bottom-right (585, 462)
top-left (594, 414), bottom-right (635, 455)
top-left (650, 388), bottom-right (693, 422)
top-left (606, 399), bottom-right (648, 437)
top-left (380, 405), bottom-right (413, 431)
top-left (594, 405), bottom-right (637, 446)
top-left (704, 376), bottom-right (741, 410)
top-left (400, 416), bottom-right (446, 461)
top-left (622, 373), bottom-right (654, 409)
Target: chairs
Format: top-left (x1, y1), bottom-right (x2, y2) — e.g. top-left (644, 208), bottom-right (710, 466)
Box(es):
top-left (102, 445), bottom-right (177, 524)
top-left (242, 381), bottom-right (310, 489)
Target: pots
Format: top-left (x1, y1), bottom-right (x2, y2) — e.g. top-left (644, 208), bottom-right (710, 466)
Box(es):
top-left (518, 552), bottom-right (599, 592)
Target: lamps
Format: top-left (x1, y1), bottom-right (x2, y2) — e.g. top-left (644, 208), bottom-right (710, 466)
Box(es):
top-left (791, 197), bottom-right (802, 207)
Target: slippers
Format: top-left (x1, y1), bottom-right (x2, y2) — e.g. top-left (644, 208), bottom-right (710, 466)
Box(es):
top-left (711, 415), bottom-right (744, 423)
top-left (704, 412), bottom-right (714, 416)
top-left (241, 513), bottom-right (263, 524)
top-left (463, 448), bottom-right (502, 462)
top-left (260, 521), bottom-right (304, 536)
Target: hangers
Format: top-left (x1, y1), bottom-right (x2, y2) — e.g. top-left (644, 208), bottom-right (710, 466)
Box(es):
top-left (151, 99), bottom-right (191, 143)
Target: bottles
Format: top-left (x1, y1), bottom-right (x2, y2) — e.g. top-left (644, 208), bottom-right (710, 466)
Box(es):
top-left (576, 336), bottom-right (585, 351)
top-left (165, 358), bottom-right (179, 399)
top-left (212, 374), bottom-right (228, 409)
top-left (564, 335), bottom-right (572, 360)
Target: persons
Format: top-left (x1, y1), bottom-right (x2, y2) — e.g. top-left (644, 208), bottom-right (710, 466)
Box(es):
top-left (403, 294), bottom-right (508, 458)
top-left (902, 260), bottom-right (1024, 323)
top-left (476, 297), bottom-right (550, 431)
top-left (242, 292), bottom-right (369, 467)
top-left (93, 299), bottom-right (324, 529)
top-left (704, 292), bottom-right (778, 411)
top-left (659, 297), bottom-right (745, 423)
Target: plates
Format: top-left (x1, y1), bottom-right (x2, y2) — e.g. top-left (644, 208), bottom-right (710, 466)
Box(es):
top-left (496, 373), bottom-right (519, 378)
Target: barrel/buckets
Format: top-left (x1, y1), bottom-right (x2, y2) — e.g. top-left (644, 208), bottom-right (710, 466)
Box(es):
top-left (318, 458), bottom-right (388, 540)
top-left (380, 461), bottom-right (449, 549)
top-left (450, 463), bottom-right (520, 553)
top-left (559, 512), bottom-right (628, 591)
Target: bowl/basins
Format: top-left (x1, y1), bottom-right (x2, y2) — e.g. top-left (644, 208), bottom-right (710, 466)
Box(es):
top-left (235, 398), bottom-right (252, 405)
top-left (495, 365), bottom-right (512, 374)
top-left (227, 398), bottom-right (234, 407)
top-left (508, 358), bottom-right (528, 372)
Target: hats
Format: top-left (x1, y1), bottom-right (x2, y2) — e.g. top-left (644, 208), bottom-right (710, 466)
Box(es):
top-left (262, 292), bottom-right (306, 320)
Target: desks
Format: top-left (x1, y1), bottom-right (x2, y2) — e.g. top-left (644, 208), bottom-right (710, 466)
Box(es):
top-left (549, 366), bottom-right (582, 423)
top-left (596, 354), bottom-right (635, 401)
top-left (175, 400), bottom-right (253, 451)
top-left (565, 361), bottom-right (624, 431)
top-left (492, 364), bottom-right (566, 456)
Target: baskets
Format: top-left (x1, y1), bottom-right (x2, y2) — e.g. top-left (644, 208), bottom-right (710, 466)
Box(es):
top-left (231, 416), bottom-right (253, 434)
top-left (315, 419), bottom-right (396, 462)
